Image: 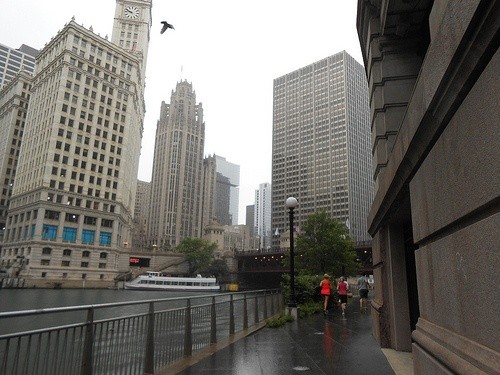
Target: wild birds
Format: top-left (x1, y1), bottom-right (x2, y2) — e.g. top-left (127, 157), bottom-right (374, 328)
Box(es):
top-left (160, 21), bottom-right (175, 34)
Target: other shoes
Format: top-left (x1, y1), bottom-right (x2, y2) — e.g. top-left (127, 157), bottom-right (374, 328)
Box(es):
top-left (320, 309), bottom-right (368, 316)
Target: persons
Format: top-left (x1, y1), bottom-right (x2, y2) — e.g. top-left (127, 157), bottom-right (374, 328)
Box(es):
top-left (357, 272), bottom-right (371, 312)
top-left (320, 274), bottom-right (333, 314)
top-left (337, 276), bottom-right (349, 315)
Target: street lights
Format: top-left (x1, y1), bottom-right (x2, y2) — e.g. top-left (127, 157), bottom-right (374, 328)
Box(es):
top-left (284, 195), bottom-right (301, 319)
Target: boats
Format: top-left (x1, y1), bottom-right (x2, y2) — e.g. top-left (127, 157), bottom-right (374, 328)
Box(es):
top-left (126, 271), bottom-right (220, 292)
